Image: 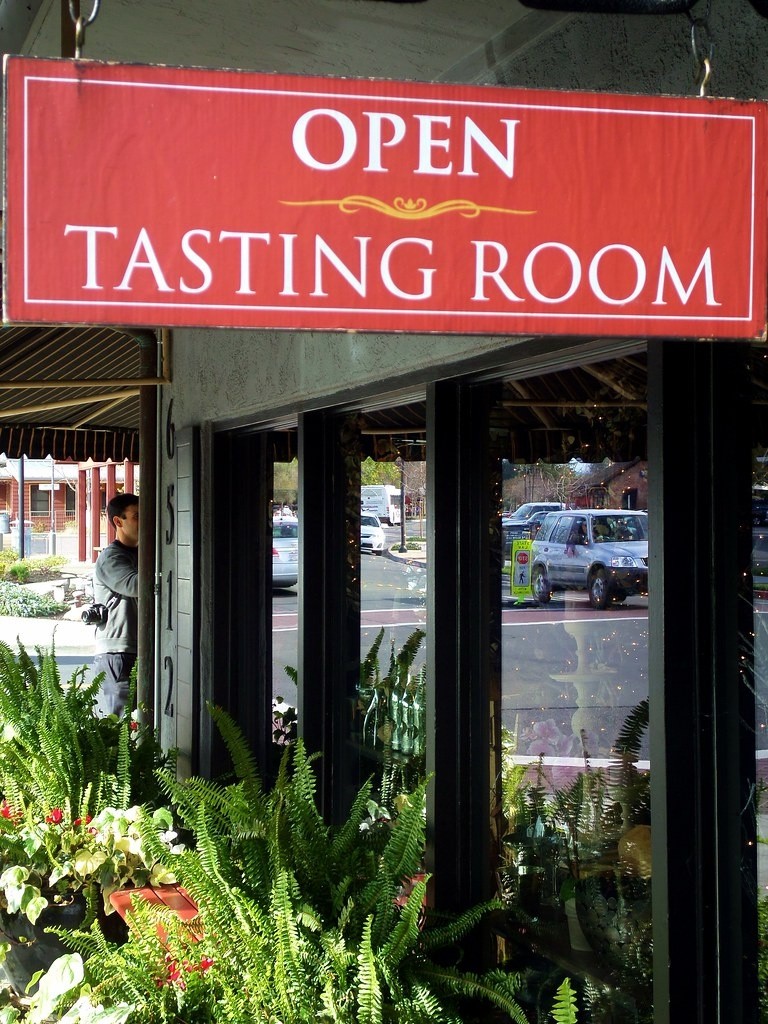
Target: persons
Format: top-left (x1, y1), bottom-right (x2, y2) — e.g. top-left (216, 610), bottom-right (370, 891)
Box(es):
top-left (580, 521), bottom-right (597, 544)
top-left (94, 494), bottom-right (139, 717)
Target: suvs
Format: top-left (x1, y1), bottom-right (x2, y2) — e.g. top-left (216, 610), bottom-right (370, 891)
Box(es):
top-left (502, 502), bottom-right (578, 561)
top-left (528, 508), bottom-right (648, 612)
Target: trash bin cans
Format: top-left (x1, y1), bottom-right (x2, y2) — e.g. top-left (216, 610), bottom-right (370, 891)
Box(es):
top-left (9, 520), bottom-right (34, 557)
top-left (0, 510), bottom-right (11, 534)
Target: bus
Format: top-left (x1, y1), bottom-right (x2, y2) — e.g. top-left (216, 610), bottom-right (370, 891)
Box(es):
top-left (361, 484), bottom-right (402, 527)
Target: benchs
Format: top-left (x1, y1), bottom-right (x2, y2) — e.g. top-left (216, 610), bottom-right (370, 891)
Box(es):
top-left (110, 876), bottom-right (223, 990)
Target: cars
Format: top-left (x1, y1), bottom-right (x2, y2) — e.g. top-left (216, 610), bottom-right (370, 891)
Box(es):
top-left (749, 526), bottom-right (768, 584)
top-left (360, 510), bottom-right (389, 556)
top-left (271, 515), bottom-right (298, 589)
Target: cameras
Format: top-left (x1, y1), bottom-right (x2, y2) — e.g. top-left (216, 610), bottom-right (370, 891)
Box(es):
top-left (81, 604), bottom-right (108, 625)
